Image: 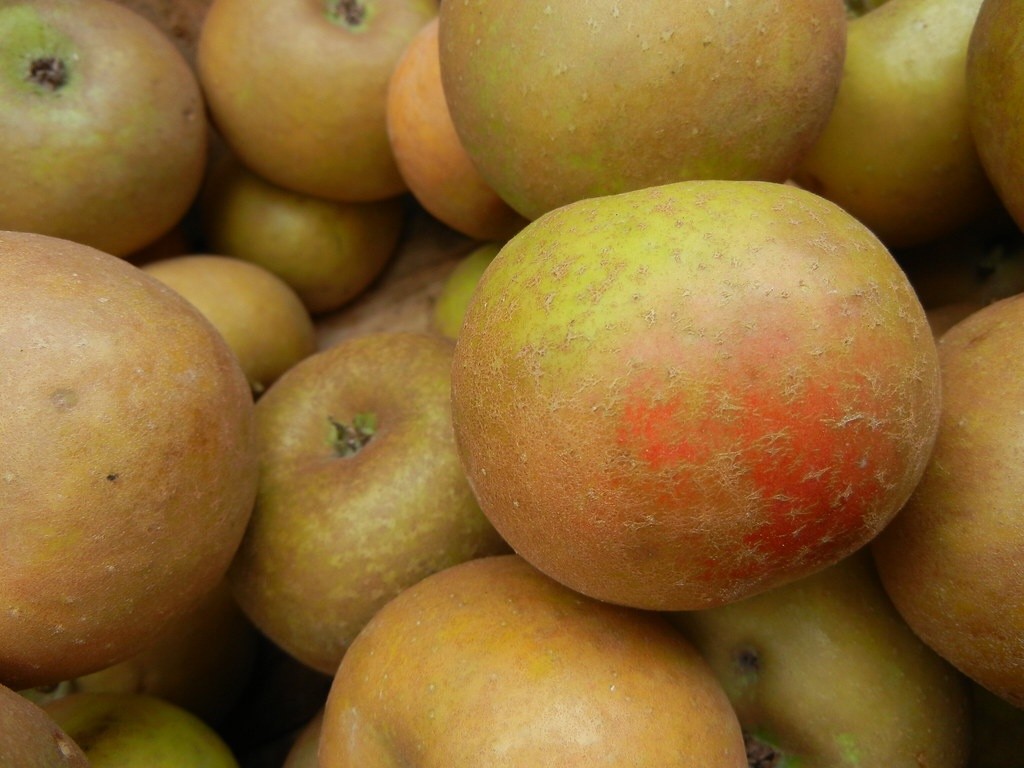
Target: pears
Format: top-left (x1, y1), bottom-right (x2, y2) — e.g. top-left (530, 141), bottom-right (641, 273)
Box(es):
top-left (0, 0), bottom-right (1024, 768)
